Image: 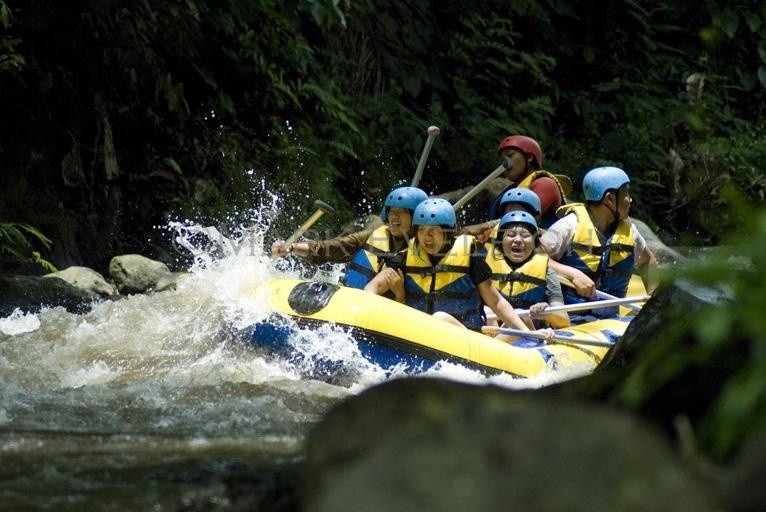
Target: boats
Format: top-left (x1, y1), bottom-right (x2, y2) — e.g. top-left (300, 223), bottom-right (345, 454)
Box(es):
top-left (218, 272), bottom-right (656, 389)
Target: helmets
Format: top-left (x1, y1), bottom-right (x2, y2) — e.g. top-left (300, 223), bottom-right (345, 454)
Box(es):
top-left (385, 187), bottom-right (429, 210)
top-left (412, 198), bottom-right (457, 230)
top-left (497, 211), bottom-right (538, 241)
top-left (498, 136), bottom-right (541, 168)
top-left (582, 167), bottom-right (631, 201)
top-left (499, 188), bottom-right (541, 216)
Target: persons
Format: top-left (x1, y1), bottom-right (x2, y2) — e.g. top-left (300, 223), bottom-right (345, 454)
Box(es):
top-left (270, 135), bottom-right (660, 344)
